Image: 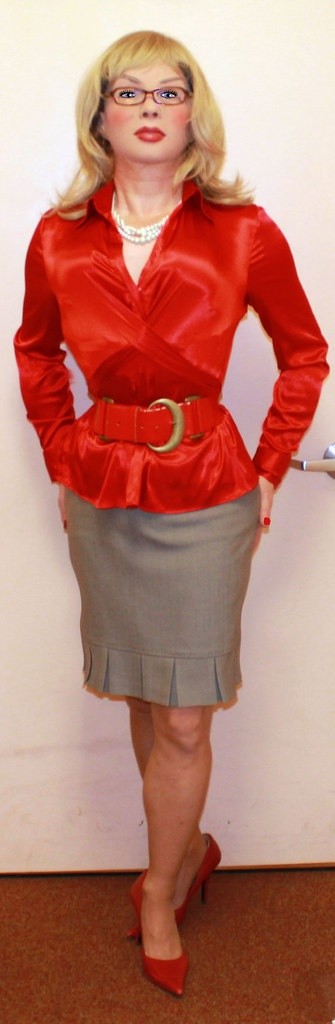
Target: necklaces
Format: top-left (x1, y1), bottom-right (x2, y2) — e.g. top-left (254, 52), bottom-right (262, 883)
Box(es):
top-left (110, 191), bottom-right (182, 244)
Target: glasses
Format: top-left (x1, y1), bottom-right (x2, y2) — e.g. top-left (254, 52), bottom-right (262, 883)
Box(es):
top-left (101, 87), bottom-right (192, 105)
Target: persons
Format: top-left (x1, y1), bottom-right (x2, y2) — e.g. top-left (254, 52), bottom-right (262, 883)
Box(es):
top-left (12, 29), bottom-right (330, 994)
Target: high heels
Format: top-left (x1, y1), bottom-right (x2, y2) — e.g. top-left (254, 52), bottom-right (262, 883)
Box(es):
top-left (123, 832), bottom-right (221, 939)
top-left (129, 870), bottom-right (188, 996)
